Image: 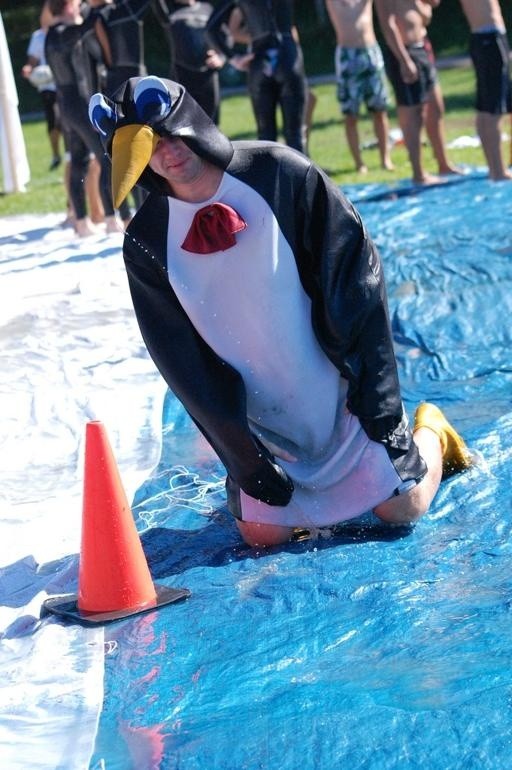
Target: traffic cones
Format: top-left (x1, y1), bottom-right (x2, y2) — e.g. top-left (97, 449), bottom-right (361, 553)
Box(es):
top-left (43, 421), bottom-right (193, 627)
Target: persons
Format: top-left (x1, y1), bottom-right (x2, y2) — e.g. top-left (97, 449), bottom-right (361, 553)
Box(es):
top-left (203, 1), bottom-right (326, 154)
top-left (462, 1), bottom-right (512, 181)
top-left (327, 2), bottom-right (395, 173)
top-left (87, 76), bottom-right (468, 548)
top-left (375, 1), bottom-right (460, 184)
top-left (22, 1), bottom-right (233, 235)
